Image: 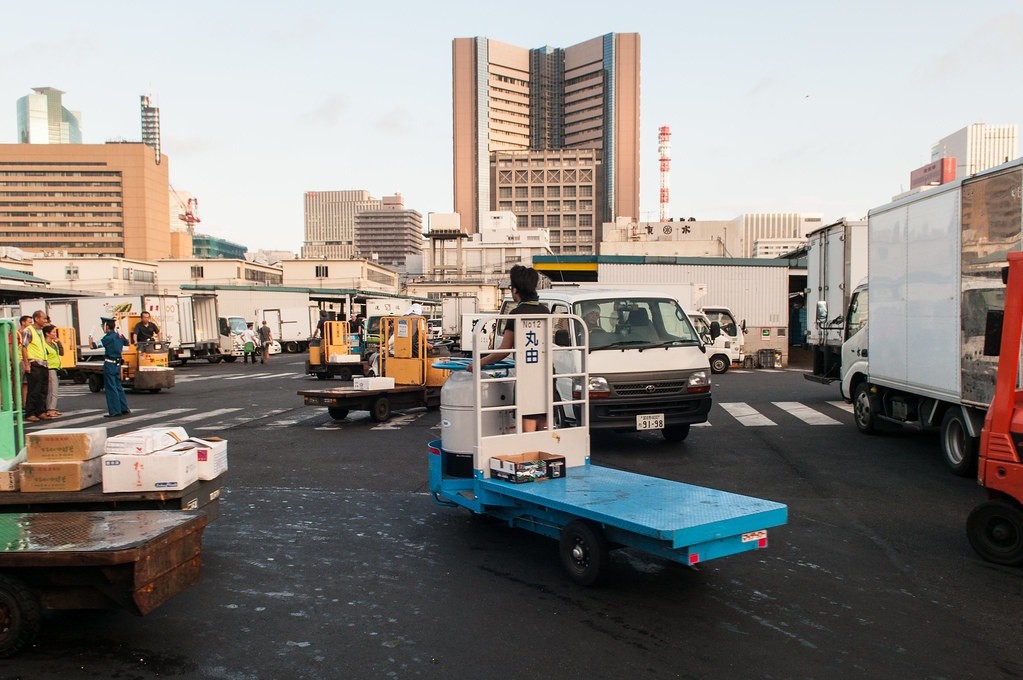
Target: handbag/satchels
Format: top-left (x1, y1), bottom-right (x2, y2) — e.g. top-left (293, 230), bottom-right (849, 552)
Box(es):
top-left (245, 342), bottom-right (254, 353)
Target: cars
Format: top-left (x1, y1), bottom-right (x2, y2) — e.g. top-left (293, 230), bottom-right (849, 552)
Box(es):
top-left (248, 322), bottom-right (282, 356)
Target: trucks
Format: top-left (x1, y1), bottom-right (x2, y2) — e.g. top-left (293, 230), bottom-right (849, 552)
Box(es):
top-left (965, 252), bottom-right (1023, 568)
top-left (441, 296), bottom-right (479, 349)
top-left (680, 307), bottom-right (748, 374)
top-left (814, 156), bottom-right (1023, 475)
top-left (180, 316), bottom-right (252, 365)
top-left (803, 217), bottom-right (868, 385)
top-left (20, 291), bottom-right (221, 373)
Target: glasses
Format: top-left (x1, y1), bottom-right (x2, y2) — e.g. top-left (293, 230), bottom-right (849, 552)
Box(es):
top-left (507, 284), bottom-right (513, 290)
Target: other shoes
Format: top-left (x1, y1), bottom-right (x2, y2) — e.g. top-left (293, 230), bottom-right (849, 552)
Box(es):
top-left (37, 413), bottom-right (52, 420)
top-left (122, 410), bottom-right (131, 414)
top-left (47, 411), bottom-right (58, 417)
top-left (103, 414), bottom-right (123, 418)
top-left (26, 416), bottom-right (41, 422)
top-left (54, 411), bottom-right (63, 415)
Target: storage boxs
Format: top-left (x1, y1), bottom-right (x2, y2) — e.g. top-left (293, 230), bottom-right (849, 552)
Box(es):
top-left (354, 377), bottom-right (395, 391)
top-left (489, 451), bottom-right (567, 485)
top-left (329, 354), bottom-right (360, 363)
top-left (0, 428), bottom-right (229, 493)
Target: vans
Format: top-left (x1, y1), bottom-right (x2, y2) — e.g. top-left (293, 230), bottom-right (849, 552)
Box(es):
top-left (494, 286), bottom-right (712, 444)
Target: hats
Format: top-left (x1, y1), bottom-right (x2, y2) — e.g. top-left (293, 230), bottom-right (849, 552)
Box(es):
top-left (582, 303), bottom-right (601, 318)
top-left (246, 322), bottom-right (254, 326)
top-left (100, 317), bottom-right (117, 325)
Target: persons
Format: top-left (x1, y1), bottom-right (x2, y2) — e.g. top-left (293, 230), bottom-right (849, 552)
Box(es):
top-left (347, 315), bottom-right (363, 342)
top-left (369, 322), bottom-right (394, 377)
top-left (240, 324), bottom-right (258, 363)
top-left (89, 317), bottom-right (131, 417)
top-left (313, 310), bottom-right (332, 355)
top-left (578, 303), bottom-right (600, 334)
top-left (467, 265), bottom-right (554, 433)
top-left (133, 311), bottom-right (161, 352)
top-left (258, 321), bottom-right (272, 364)
top-left (8, 311), bottom-right (64, 421)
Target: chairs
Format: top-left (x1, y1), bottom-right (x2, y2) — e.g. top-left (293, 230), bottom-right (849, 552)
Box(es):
top-left (629, 308), bottom-right (658, 342)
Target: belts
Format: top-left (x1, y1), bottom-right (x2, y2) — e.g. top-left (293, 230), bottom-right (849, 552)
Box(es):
top-left (105, 358), bottom-right (116, 363)
